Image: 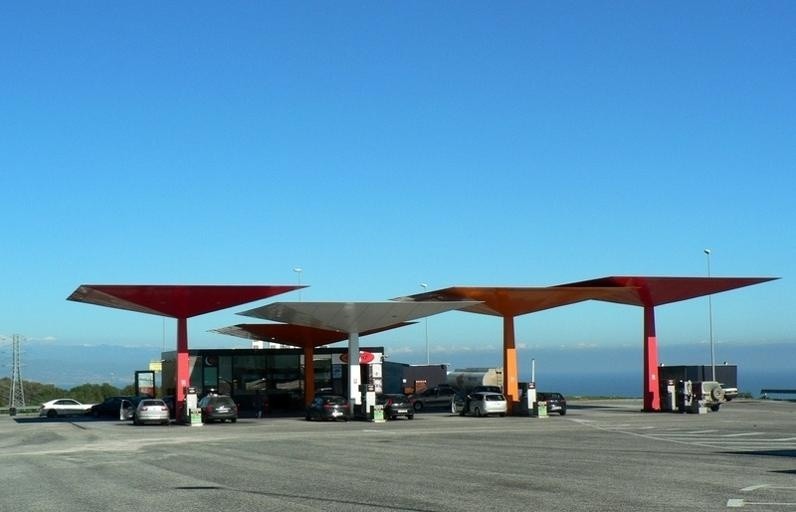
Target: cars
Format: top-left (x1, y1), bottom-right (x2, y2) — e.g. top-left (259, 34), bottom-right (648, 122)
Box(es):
top-left (407, 384), bottom-right (508, 417)
top-left (39, 397), bottom-right (171, 425)
top-left (198, 393), bottom-right (238, 423)
top-left (376, 393), bottom-right (415, 420)
top-left (304, 395), bottom-right (352, 422)
top-left (536, 392), bottom-right (566, 416)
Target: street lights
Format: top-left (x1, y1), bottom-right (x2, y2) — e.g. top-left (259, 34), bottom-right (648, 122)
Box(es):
top-left (294, 268), bottom-right (302, 303)
top-left (704, 249), bottom-right (715, 381)
top-left (420, 282), bottom-right (429, 364)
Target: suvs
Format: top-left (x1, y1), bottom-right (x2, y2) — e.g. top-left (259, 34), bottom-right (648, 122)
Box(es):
top-left (692, 380), bottom-right (725, 411)
